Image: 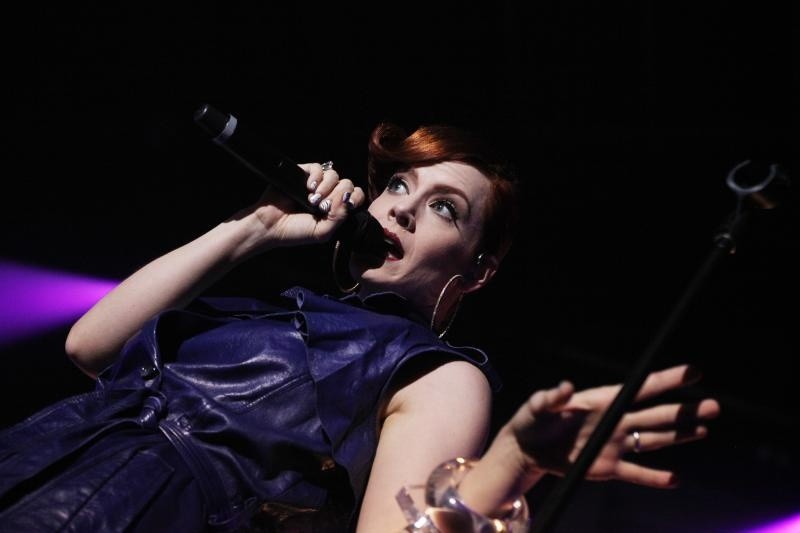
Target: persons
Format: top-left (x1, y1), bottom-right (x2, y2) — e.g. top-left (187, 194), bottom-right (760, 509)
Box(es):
top-left (0, 120), bottom-right (719, 533)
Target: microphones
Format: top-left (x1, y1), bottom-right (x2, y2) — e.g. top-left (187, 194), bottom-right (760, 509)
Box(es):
top-left (193, 103), bottom-right (386, 254)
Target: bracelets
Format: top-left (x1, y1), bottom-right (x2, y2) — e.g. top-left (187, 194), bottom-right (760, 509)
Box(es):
top-left (396, 455), bottom-right (529, 533)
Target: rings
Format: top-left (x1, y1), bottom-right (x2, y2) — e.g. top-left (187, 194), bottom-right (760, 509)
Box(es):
top-left (632, 431), bottom-right (640, 453)
